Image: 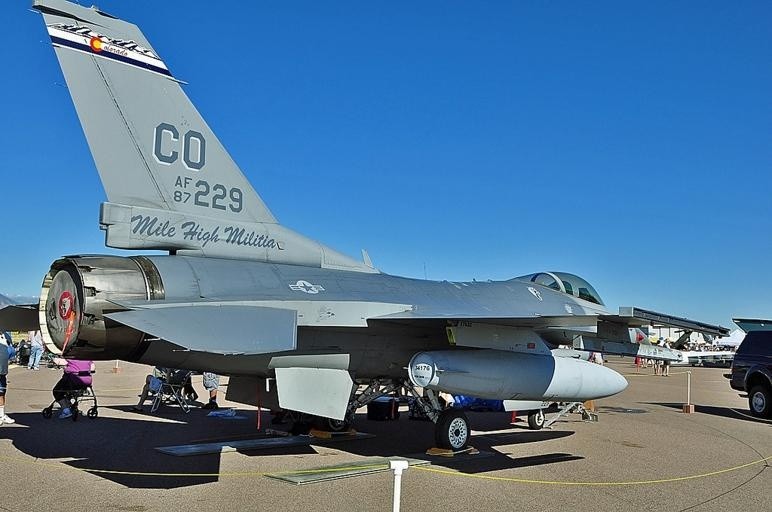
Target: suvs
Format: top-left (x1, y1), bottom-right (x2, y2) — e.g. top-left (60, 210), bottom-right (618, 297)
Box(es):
top-left (730, 319), bottom-right (772, 419)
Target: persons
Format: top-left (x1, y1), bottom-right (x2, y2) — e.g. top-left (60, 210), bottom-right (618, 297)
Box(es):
top-left (201, 371), bottom-right (219, 410)
top-left (133, 368), bottom-right (190, 412)
top-left (588, 351), bottom-right (603, 364)
top-left (27, 330), bottom-right (44, 371)
top-left (52, 359), bottom-right (97, 419)
top-left (634, 337), bottom-right (734, 376)
top-left (0, 332), bottom-right (16, 427)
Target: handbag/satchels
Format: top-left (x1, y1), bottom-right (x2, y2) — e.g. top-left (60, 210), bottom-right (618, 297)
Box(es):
top-left (407, 396), bottom-right (446, 422)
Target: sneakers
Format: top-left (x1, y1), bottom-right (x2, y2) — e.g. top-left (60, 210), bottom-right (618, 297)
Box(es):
top-left (59, 408), bottom-right (73, 419)
top-left (203, 403), bottom-right (218, 409)
top-left (0, 414), bottom-right (15, 427)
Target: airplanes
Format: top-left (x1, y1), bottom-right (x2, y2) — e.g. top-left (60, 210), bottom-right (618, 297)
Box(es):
top-left (1, 0), bottom-right (690, 452)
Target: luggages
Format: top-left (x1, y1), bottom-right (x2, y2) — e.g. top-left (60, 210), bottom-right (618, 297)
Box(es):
top-left (368, 397), bottom-right (399, 421)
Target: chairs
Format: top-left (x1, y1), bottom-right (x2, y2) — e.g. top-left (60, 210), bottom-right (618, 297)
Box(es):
top-left (150, 372), bottom-right (193, 414)
top-left (40, 370), bottom-right (98, 421)
